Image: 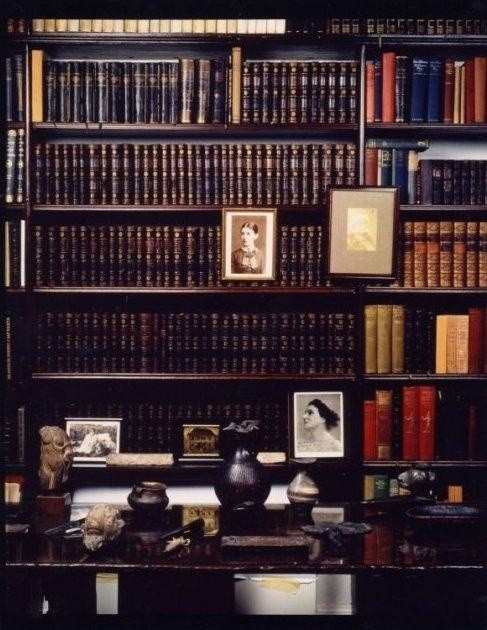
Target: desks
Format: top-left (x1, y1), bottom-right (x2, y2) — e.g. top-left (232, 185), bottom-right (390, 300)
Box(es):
top-left (0, 507), bottom-right (484, 628)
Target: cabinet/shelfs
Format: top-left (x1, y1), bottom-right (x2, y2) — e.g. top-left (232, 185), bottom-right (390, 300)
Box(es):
top-left (350, 29), bottom-right (482, 514)
top-left (0, 1), bottom-right (371, 514)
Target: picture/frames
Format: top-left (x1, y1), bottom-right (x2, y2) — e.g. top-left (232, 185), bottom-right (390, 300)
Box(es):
top-left (318, 180), bottom-right (401, 284)
top-left (284, 388), bottom-right (348, 462)
top-left (218, 203), bottom-right (277, 282)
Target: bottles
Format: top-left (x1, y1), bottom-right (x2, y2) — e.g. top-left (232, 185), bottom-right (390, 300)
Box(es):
top-left (127, 480), bottom-right (169, 520)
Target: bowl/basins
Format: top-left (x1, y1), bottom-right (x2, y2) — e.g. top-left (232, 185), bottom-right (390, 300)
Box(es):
top-left (405, 506), bottom-right (483, 538)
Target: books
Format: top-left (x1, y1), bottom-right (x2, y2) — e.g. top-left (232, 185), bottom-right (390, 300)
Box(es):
top-left (360, 503), bottom-right (440, 569)
